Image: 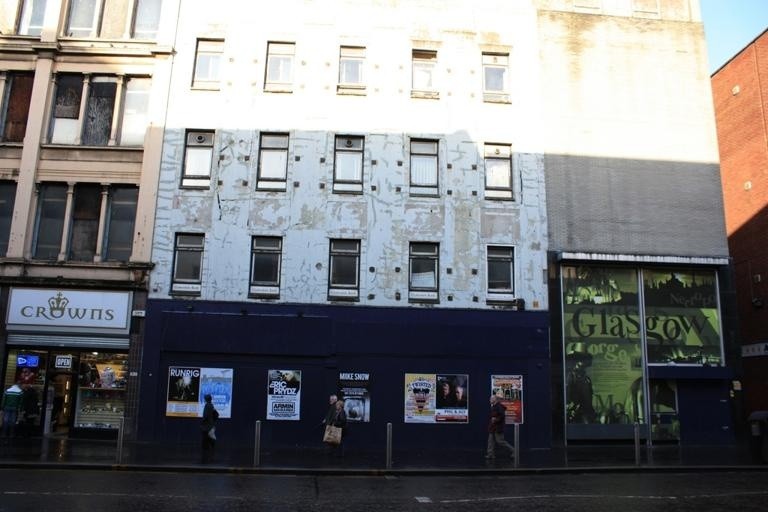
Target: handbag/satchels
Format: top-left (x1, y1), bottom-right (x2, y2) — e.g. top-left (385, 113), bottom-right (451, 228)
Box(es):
top-left (212, 410), bottom-right (219, 421)
top-left (322, 421), bottom-right (343, 446)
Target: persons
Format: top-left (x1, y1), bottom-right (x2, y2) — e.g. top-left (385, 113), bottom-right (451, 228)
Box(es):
top-left (484, 394), bottom-right (515, 462)
top-left (200, 394), bottom-right (219, 462)
top-left (21, 385), bottom-right (42, 434)
top-left (1, 384), bottom-right (24, 438)
top-left (281, 371), bottom-right (300, 383)
top-left (329, 400), bottom-right (346, 465)
top-left (449, 386), bottom-right (467, 409)
top-left (440, 384), bottom-right (451, 403)
top-left (322, 394), bottom-right (338, 426)
